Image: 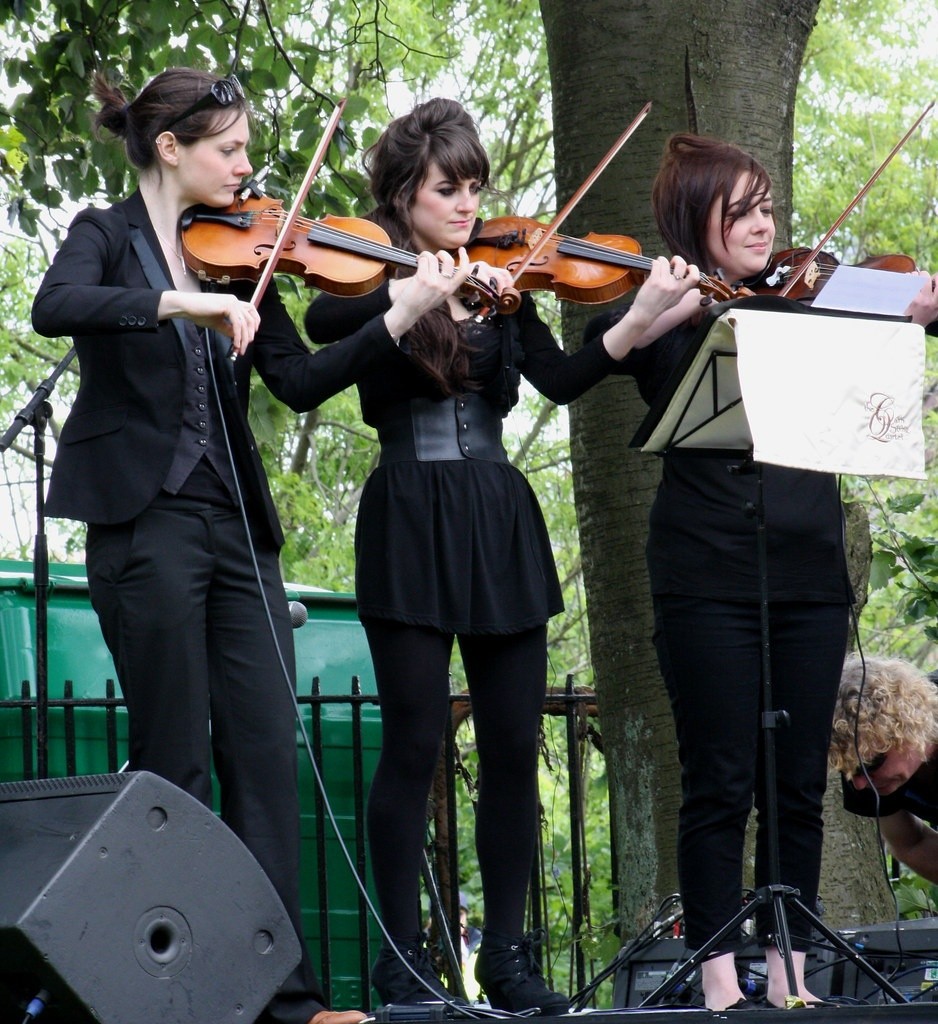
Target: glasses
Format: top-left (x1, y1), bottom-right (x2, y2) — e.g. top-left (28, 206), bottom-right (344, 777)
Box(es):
top-left (855, 751), bottom-right (885, 777)
top-left (164, 73), bottom-right (246, 132)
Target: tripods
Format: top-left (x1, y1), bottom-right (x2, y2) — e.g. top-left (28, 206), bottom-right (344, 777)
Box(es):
top-left (631, 320), bottom-right (919, 1006)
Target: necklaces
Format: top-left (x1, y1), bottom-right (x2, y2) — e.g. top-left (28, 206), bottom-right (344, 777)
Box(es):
top-left (155, 228), bottom-right (187, 276)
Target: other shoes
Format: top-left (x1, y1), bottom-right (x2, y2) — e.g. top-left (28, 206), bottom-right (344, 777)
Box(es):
top-left (308, 1010), bottom-right (367, 1024)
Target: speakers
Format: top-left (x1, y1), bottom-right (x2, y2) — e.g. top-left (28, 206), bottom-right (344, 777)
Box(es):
top-left (0, 771), bottom-right (302, 1023)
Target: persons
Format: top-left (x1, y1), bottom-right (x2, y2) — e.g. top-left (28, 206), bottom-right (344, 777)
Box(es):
top-left (828, 652), bottom-right (938, 882)
top-left (303, 97), bottom-right (703, 1016)
top-left (32, 66), bottom-right (471, 1024)
top-left (583, 134), bottom-right (938, 1024)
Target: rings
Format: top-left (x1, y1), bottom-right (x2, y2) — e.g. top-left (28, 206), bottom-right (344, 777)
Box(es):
top-left (441, 270), bottom-right (452, 279)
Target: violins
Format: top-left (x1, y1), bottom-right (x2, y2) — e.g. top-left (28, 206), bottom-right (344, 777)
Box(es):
top-left (737, 244), bottom-right (936, 323)
top-left (448, 213), bottom-right (758, 310)
top-left (179, 194), bottom-right (523, 324)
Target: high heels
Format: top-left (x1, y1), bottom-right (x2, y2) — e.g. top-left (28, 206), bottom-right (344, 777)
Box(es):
top-left (474, 926), bottom-right (570, 1015)
top-left (372, 933), bottom-right (469, 1010)
